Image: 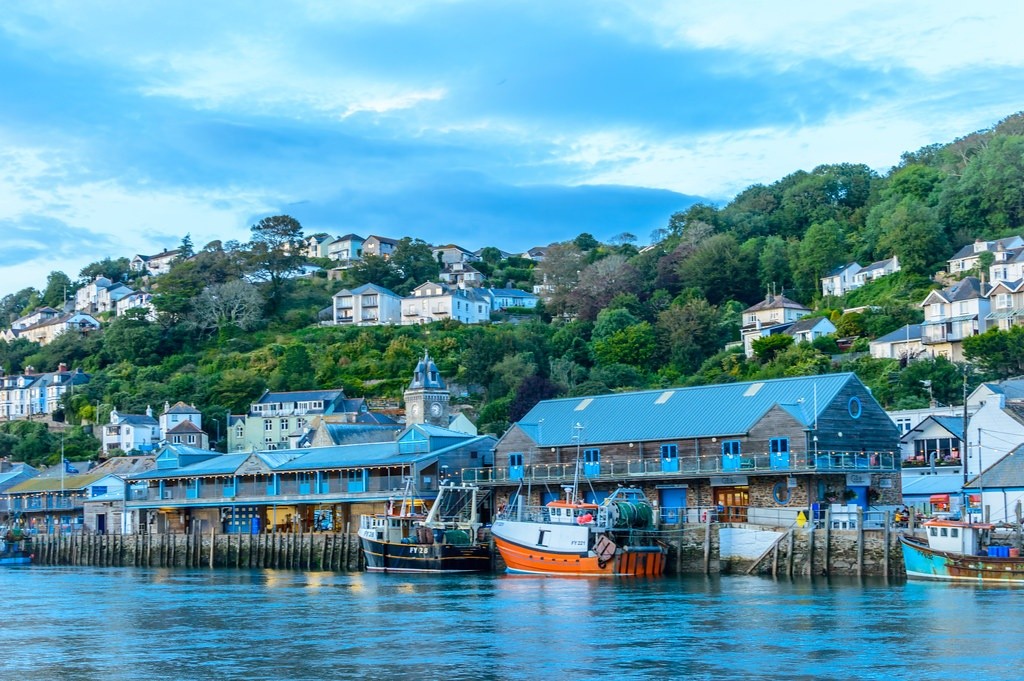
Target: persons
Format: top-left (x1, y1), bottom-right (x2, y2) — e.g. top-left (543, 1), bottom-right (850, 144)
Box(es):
top-left (284, 513), bottom-right (292, 533)
top-left (895, 506), bottom-right (922, 528)
top-left (977, 546), bottom-right (988, 556)
top-left (314, 513), bottom-right (325, 533)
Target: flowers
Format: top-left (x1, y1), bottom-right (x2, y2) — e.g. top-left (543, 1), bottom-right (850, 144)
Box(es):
top-left (943, 455), bottom-right (957, 463)
top-left (868, 487), bottom-right (881, 502)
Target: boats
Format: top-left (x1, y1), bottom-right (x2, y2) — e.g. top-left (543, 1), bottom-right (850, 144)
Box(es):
top-left (356, 473), bottom-right (495, 575)
top-left (487, 422), bottom-right (669, 579)
top-left (0, 513), bottom-right (35, 565)
top-left (896, 514), bottom-right (1024, 585)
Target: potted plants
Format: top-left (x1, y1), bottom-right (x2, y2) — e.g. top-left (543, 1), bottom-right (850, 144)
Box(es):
top-left (823, 491), bottom-right (840, 504)
top-left (934, 459), bottom-right (942, 467)
top-left (842, 489), bottom-right (855, 501)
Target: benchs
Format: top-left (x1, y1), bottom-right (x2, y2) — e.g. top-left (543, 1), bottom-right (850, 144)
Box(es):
top-left (906, 521), bottom-right (921, 528)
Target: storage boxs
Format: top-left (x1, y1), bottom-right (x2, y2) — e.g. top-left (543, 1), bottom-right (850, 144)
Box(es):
top-left (988, 546), bottom-right (1015, 558)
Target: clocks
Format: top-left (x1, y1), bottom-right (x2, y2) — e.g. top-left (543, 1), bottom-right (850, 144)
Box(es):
top-left (431, 404), bottom-right (440, 416)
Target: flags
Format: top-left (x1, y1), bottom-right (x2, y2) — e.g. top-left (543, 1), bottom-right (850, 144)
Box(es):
top-left (64, 459), bottom-right (79, 473)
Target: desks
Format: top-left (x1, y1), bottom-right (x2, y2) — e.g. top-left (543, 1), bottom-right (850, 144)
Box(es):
top-left (901, 516), bottom-right (924, 528)
